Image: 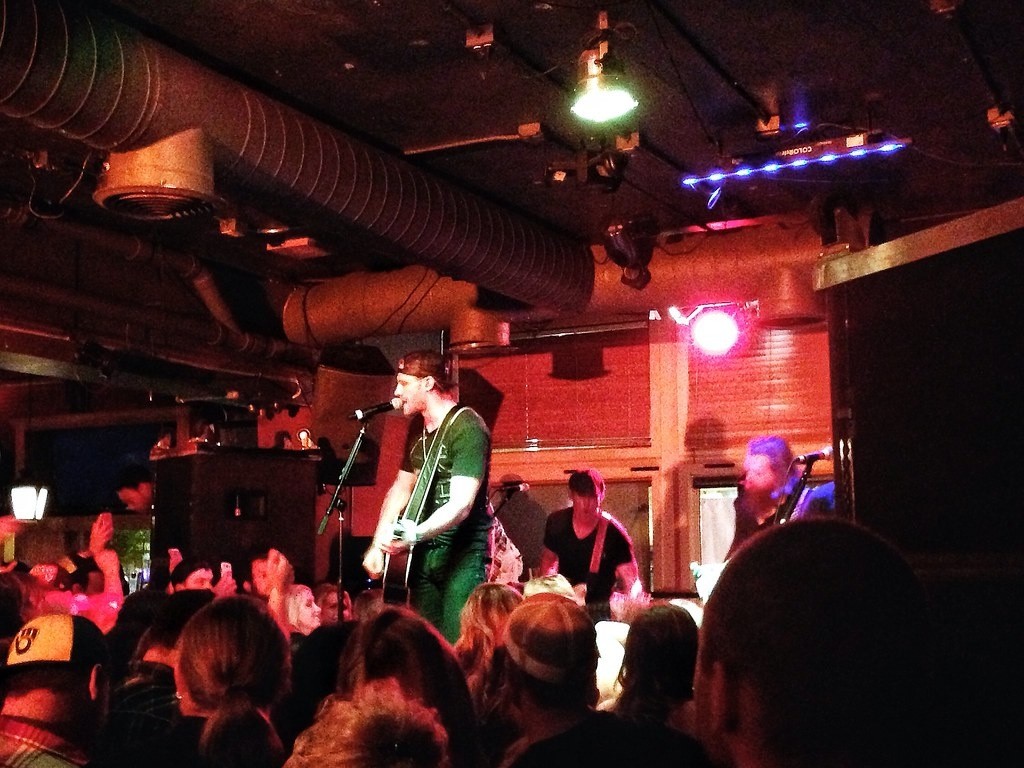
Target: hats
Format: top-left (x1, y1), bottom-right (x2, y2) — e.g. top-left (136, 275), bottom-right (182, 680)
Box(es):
top-left (502, 592), bottom-right (597, 682)
top-left (399, 350), bottom-right (457, 386)
top-left (6, 615), bottom-right (113, 676)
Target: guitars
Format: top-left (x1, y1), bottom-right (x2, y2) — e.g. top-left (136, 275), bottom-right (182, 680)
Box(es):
top-left (381, 515), bottom-right (415, 607)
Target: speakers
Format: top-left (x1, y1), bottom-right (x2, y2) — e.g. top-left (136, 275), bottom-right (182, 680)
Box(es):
top-left (150, 452), bottom-right (315, 598)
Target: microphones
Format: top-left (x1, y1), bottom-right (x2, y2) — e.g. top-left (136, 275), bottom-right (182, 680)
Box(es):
top-left (497, 482), bottom-right (530, 492)
top-left (348, 397), bottom-right (403, 420)
top-left (795, 446), bottom-right (834, 464)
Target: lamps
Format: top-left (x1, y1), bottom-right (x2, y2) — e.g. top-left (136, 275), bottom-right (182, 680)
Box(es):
top-left (572, 49), bottom-right (638, 124)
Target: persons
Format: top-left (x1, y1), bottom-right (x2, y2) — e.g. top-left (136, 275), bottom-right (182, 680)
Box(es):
top-left (114, 465), bottom-right (154, 508)
top-left (601, 606), bottom-right (699, 731)
top-left (502, 594), bottom-right (723, 768)
top-left (693, 519), bottom-right (928, 768)
top-left (1, 514), bottom-right (471, 768)
top-left (741, 437), bottom-right (834, 526)
top-left (150, 430), bottom-right (172, 461)
top-left (540, 468), bottom-right (636, 603)
top-left (455, 583), bottom-right (524, 714)
top-left (362, 353), bottom-right (491, 640)
